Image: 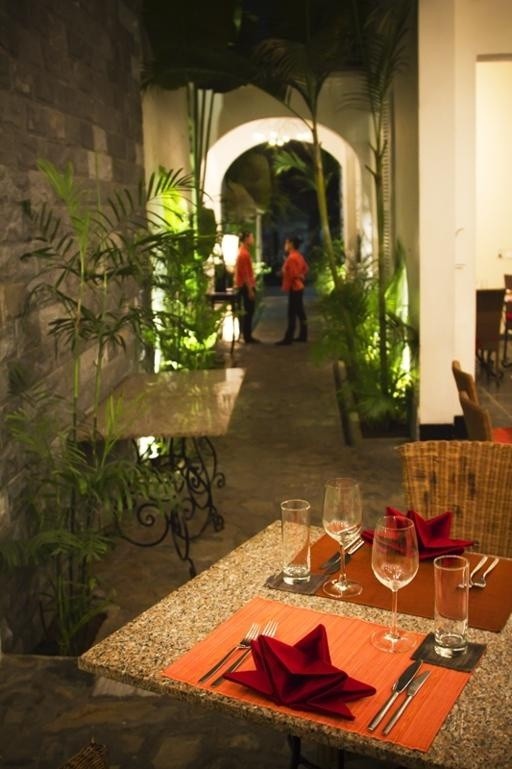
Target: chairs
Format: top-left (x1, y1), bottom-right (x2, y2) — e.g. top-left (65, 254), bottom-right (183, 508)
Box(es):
top-left (459, 390), bottom-right (493, 445)
top-left (475, 286), bottom-right (506, 387)
top-left (396, 438), bottom-right (511, 558)
top-left (452, 361), bottom-right (512, 444)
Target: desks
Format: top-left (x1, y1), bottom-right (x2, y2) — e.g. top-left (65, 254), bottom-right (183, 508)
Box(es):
top-left (68, 366), bottom-right (248, 579)
top-left (204, 287), bottom-right (246, 358)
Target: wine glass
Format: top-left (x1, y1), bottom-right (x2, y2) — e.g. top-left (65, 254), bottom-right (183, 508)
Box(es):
top-left (372, 518), bottom-right (420, 654)
top-left (324, 481), bottom-right (363, 599)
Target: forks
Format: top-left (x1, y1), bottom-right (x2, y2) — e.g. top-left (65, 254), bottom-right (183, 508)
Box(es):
top-left (199, 622), bottom-right (286, 689)
top-left (458, 554), bottom-right (499, 589)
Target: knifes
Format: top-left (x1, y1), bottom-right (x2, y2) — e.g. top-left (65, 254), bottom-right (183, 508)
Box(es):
top-left (322, 533), bottom-right (364, 586)
top-left (370, 660), bottom-right (430, 733)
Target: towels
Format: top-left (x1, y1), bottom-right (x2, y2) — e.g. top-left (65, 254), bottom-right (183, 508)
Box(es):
top-left (359, 505), bottom-right (473, 560)
top-left (222, 627), bottom-right (375, 721)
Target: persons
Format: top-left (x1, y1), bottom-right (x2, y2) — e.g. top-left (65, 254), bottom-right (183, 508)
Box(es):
top-left (275, 236), bottom-right (309, 347)
top-left (232, 230), bottom-right (260, 344)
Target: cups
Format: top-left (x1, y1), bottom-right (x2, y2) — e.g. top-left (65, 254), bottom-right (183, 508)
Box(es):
top-left (433, 556), bottom-right (470, 659)
top-left (281, 500), bottom-right (312, 586)
